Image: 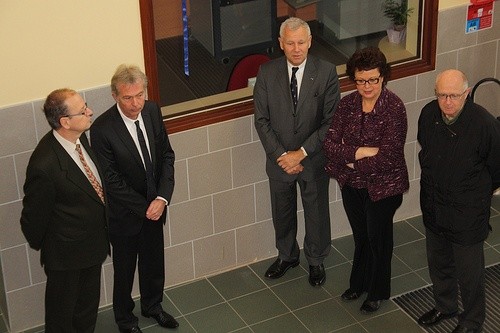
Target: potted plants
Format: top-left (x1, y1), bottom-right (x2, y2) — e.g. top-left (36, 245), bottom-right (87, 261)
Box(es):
top-left (381, 0), bottom-right (415, 46)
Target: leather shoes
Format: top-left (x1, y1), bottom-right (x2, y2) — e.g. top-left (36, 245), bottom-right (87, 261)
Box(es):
top-left (308, 263), bottom-right (327, 288)
top-left (360, 295), bottom-right (382, 313)
top-left (265, 258), bottom-right (300, 279)
top-left (141, 306), bottom-right (179, 329)
top-left (418, 307), bottom-right (458, 328)
top-left (452, 324), bottom-right (482, 333)
top-left (341, 287), bottom-right (367, 301)
top-left (118, 321), bottom-right (143, 333)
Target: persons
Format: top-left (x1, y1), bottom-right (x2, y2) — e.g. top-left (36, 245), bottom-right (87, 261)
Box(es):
top-left (417, 70), bottom-right (500, 333)
top-left (324, 46), bottom-right (409, 313)
top-left (20, 88), bottom-right (111, 333)
top-left (254, 18), bottom-right (341, 287)
top-left (90, 64), bottom-right (179, 333)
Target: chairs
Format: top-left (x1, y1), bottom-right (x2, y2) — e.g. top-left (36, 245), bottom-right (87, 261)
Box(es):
top-left (472, 77), bottom-right (500, 234)
top-left (225, 52), bottom-right (273, 92)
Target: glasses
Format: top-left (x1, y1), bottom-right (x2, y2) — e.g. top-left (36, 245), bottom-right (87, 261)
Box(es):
top-left (353, 73), bottom-right (382, 85)
top-left (65, 102), bottom-right (88, 118)
top-left (435, 88), bottom-right (468, 99)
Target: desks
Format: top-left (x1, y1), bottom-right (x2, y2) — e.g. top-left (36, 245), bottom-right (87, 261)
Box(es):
top-left (378, 35), bottom-right (416, 66)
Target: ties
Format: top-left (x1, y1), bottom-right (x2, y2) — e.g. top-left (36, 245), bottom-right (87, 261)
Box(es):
top-left (134, 119), bottom-right (153, 178)
top-left (290, 67), bottom-right (299, 113)
top-left (75, 144), bottom-right (105, 205)
